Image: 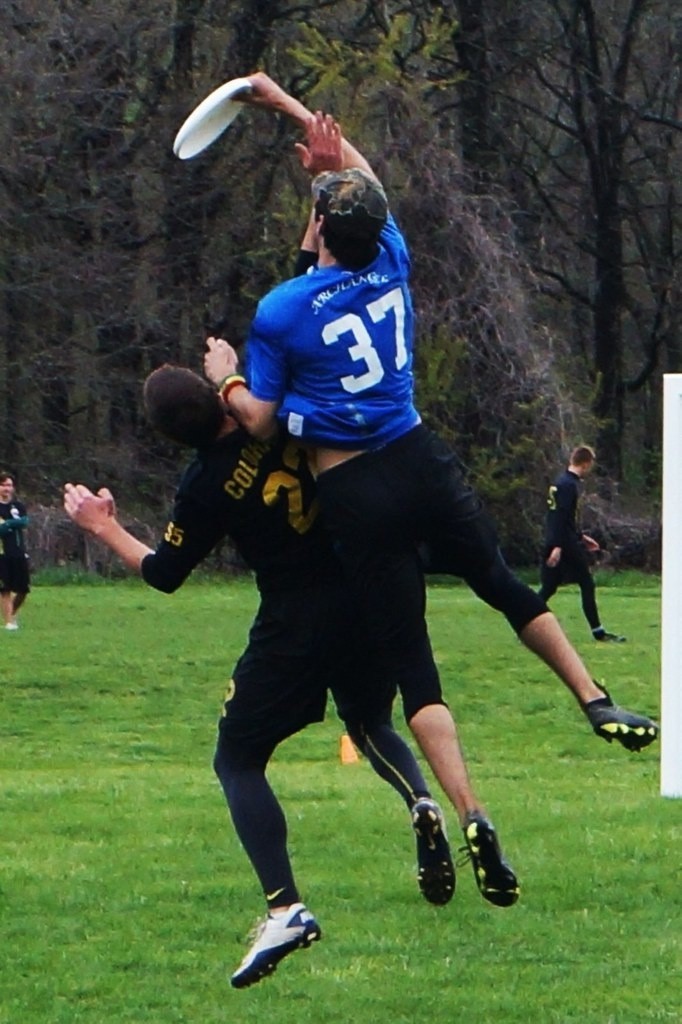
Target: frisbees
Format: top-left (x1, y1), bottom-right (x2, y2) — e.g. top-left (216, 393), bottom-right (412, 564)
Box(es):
top-left (170, 76), bottom-right (253, 160)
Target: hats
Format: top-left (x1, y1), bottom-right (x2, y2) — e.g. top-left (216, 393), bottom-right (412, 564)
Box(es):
top-left (310, 167), bottom-right (387, 242)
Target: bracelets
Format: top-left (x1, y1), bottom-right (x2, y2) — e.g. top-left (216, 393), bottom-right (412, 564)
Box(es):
top-left (218, 374), bottom-right (246, 405)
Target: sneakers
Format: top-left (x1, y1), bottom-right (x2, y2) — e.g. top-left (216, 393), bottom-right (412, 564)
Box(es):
top-left (456, 814), bottom-right (519, 908)
top-left (588, 702), bottom-right (658, 752)
top-left (410, 797), bottom-right (457, 906)
top-left (229, 902), bottom-right (322, 989)
top-left (593, 630), bottom-right (626, 643)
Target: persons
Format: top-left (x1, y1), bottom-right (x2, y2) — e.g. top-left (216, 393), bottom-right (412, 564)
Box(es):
top-left (63, 109), bottom-right (456, 991)
top-left (538, 447), bottom-right (626, 642)
top-left (201, 70), bottom-right (657, 907)
top-left (0, 472), bottom-right (31, 630)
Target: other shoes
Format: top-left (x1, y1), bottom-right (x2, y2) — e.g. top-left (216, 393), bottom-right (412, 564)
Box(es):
top-left (4, 623), bottom-right (18, 631)
